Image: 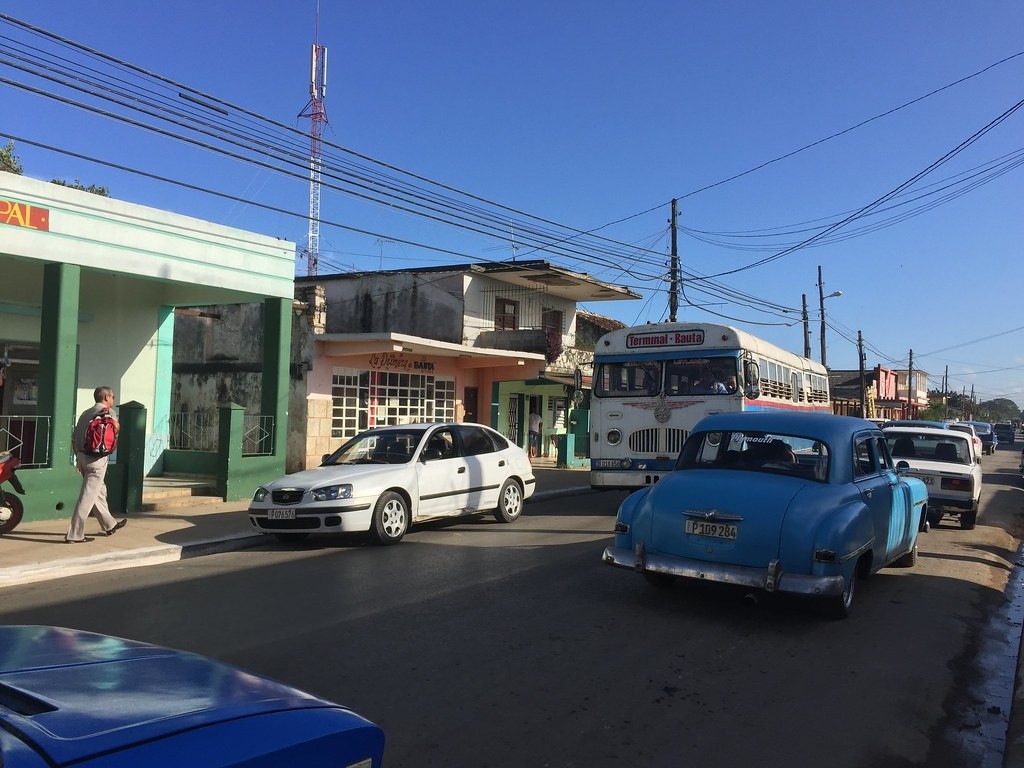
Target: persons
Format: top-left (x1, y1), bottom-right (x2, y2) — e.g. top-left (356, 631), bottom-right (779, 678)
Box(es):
top-left (1021, 450), bottom-right (1024, 478)
top-left (66, 386), bottom-right (127, 543)
top-left (646, 369), bottom-right (659, 389)
top-left (692, 367), bottom-right (736, 394)
top-left (530, 407), bottom-right (543, 458)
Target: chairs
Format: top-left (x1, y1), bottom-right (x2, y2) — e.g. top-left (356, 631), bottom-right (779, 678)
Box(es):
top-left (891, 438), bottom-right (916, 458)
top-left (934, 442), bottom-right (958, 462)
top-left (464, 437), bottom-right (487, 456)
top-left (426, 438), bottom-right (447, 460)
top-left (387, 442), bottom-right (409, 464)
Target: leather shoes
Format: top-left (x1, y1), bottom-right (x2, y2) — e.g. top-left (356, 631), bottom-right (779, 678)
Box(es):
top-left (65, 537), bottom-right (96, 545)
top-left (106, 518), bottom-right (128, 535)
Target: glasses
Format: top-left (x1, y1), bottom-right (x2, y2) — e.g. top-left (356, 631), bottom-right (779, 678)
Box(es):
top-left (108, 395), bottom-right (115, 400)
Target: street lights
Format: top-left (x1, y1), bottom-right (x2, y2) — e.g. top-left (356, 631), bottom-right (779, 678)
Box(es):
top-left (820, 291), bottom-right (842, 368)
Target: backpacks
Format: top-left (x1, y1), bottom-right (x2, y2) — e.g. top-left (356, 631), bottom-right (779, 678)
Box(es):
top-left (82, 408), bottom-right (118, 458)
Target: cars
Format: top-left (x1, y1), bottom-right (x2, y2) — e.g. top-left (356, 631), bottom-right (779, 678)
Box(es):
top-left (603, 413), bottom-right (929, 618)
top-left (881, 427), bottom-right (983, 530)
top-left (248, 422), bottom-right (536, 546)
top-left (0, 625), bottom-right (384, 768)
top-left (864, 419), bottom-right (1015, 464)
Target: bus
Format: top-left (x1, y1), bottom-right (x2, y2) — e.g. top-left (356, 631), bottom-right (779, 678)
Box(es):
top-left (573, 320), bottom-right (830, 491)
